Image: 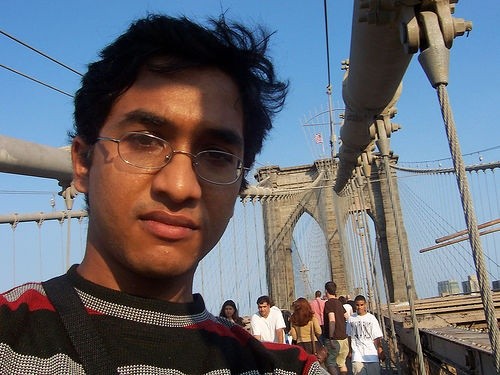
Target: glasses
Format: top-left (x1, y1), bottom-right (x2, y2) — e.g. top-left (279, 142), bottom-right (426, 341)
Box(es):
top-left (85, 132), bottom-right (251, 184)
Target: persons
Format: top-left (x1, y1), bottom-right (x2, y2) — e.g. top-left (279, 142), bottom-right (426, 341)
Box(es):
top-left (219, 300), bottom-right (246, 334)
top-left (1, 12), bottom-right (341, 375)
top-left (290, 298), bottom-right (322, 362)
top-left (309, 290), bottom-right (327, 349)
top-left (347, 295), bottom-right (386, 375)
top-left (323, 282), bottom-right (350, 375)
top-left (338, 295), bottom-right (356, 319)
top-left (250, 296), bottom-right (287, 344)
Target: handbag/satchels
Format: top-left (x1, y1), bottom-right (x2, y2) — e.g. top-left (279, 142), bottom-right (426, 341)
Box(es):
top-left (314, 346), bottom-right (329, 365)
